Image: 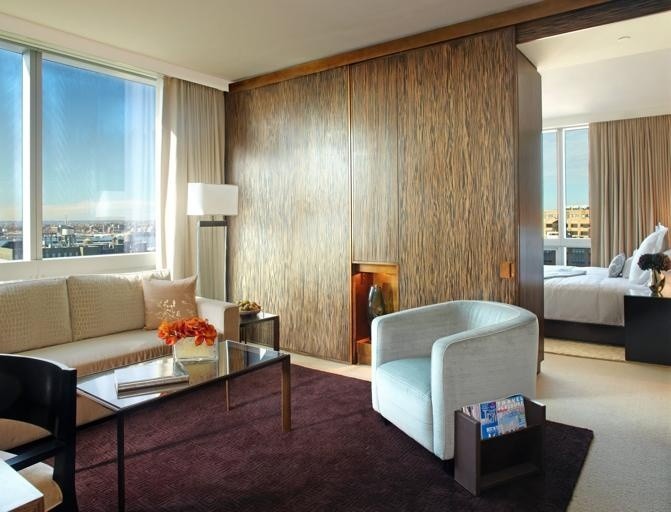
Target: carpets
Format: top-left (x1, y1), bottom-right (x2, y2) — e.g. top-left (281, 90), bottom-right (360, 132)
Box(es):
top-left (5, 347), bottom-right (595, 512)
top-left (543, 337), bottom-right (626, 362)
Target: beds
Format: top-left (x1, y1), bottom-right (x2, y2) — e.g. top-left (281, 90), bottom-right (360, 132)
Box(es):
top-left (543, 265), bottom-right (671, 346)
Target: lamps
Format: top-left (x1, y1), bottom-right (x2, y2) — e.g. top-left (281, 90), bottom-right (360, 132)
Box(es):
top-left (187, 182), bottom-right (238, 302)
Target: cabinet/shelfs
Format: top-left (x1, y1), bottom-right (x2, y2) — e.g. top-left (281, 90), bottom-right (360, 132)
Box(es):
top-left (454, 394), bottom-right (546, 497)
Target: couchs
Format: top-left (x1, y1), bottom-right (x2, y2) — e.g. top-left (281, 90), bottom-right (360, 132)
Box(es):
top-left (0, 269), bottom-right (241, 451)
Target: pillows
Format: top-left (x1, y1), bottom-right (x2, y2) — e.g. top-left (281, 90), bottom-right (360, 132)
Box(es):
top-left (142, 273), bottom-right (199, 331)
top-left (608, 222), bottom-right (671, 285)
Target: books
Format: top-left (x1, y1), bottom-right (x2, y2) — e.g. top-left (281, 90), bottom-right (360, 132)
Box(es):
top-left (114, 360), bottom-right (190, 392)
top-left (461, 392), bottom-right (527, 440)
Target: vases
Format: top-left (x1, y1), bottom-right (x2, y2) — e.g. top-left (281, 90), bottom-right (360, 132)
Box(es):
top-left (172, 335), bottom-right (220, 364)
top-left (650, 269), bottom-right (665, 292)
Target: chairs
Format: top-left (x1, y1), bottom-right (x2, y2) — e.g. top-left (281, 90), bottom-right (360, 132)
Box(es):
top-left (370, 300), bottom-right (539, 460)
top-left (0, 355), bottom-right (77, 512)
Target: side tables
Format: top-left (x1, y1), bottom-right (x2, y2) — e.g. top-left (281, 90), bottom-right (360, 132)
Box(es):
top-left (240, 311), bottom-right (280, 351)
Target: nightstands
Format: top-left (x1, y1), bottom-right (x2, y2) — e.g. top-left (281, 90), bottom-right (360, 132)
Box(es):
top-left (624, 288), bottom-right (671, 366)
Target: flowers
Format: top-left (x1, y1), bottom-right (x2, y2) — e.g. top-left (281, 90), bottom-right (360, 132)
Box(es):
top-left (155, 295), bottom-right (218, 346)
